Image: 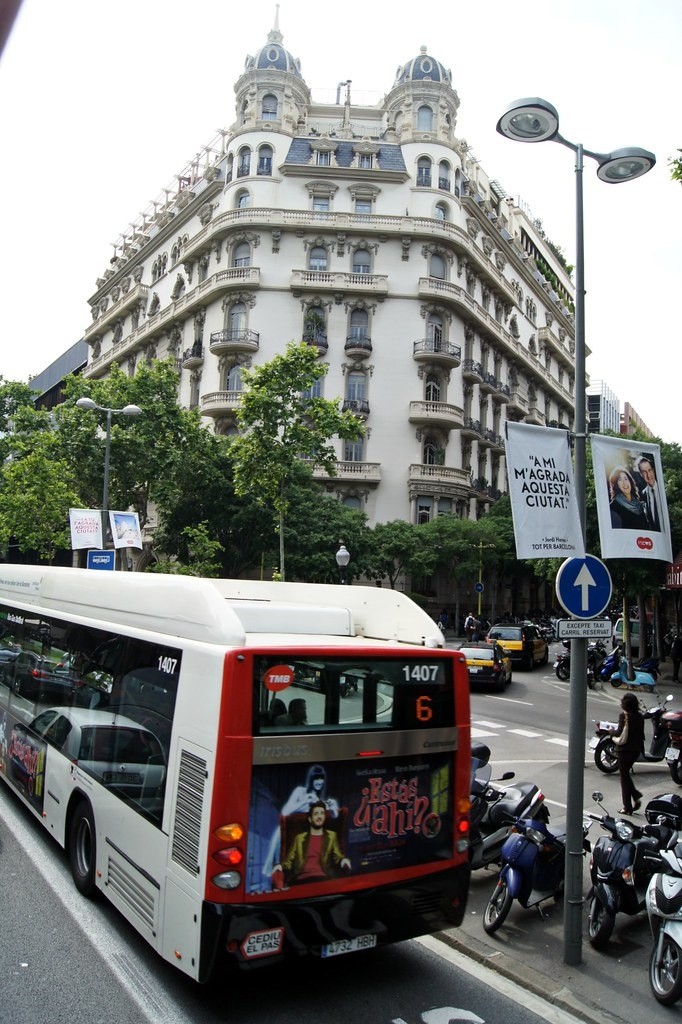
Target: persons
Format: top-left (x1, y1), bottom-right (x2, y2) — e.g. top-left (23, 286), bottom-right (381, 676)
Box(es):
top-left (438, 617), bottom-right (449, 633)
top-left (465, 612), bottom-right (480, 639)
top-left (609, 458), bottom-right (664, 528)
top-left (284, 801), bottom-right (352, 883)
top-left (614, 693), bottom-right (646, 814)
top-left (270, 698), bottom-right (306, 726)
top-left (6, 731), bottom-right (46, 791)
top-left (262, 767), bottom-right (341, 877)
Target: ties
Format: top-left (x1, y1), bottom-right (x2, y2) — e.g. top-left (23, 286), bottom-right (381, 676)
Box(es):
top-left (651, 488), bottom-right (661, 532)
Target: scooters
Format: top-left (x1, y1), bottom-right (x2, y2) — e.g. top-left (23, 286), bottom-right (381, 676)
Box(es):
top-left (601, 645), bottom-right (656, 692)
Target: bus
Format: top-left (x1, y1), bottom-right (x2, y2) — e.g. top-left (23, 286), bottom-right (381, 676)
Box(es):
top-left (0, 561), bottom-right (471, 989)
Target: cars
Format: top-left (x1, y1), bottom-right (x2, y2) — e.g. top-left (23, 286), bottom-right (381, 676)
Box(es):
top-left (458, 639), bottom-right (512, 692)
top-left (291, 664), bottom-right (358, 698)
top-left (528, 621), bottom-right (554, 642)
top-left (0, 642), bottom-right (22, 669)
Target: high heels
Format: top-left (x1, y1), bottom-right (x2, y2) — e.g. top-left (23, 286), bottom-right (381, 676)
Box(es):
top-left (617, 807), bottom-right (633, 816)
top-left (633, 800), bottom-right (641, 811)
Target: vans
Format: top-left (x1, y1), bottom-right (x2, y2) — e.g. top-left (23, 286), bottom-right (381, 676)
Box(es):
top-left (612, 619), bottom-right (641, 652)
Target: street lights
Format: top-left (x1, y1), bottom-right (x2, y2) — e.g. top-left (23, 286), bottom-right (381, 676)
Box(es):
top-left (469, 543), bottom-right (495, 640)
top-left (496, 98), bottom-right (657, 964)
top-left (77, 398), bottom-right (143, 547)
top-left (335, 547), bottom-right (352, 583)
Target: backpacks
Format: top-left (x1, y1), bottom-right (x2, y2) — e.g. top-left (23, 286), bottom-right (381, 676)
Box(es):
top-left (467, 618), bottom-right (474, 628)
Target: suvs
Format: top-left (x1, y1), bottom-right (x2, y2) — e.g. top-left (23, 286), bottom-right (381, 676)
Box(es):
top-left (0, 645), bottom-right (82, 703)
top-left (487, 622), bottom-right (549, 672)
top-left (12, 706), bottom-right (166, 816)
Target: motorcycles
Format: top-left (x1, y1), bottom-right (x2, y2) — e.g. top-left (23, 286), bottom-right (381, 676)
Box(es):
top-left (471, 742), bottom-right (682, 1006)
top-left (592, 695), bottom-right (682, 786)
top-left (554, 638), bottom-right (608, 689)
